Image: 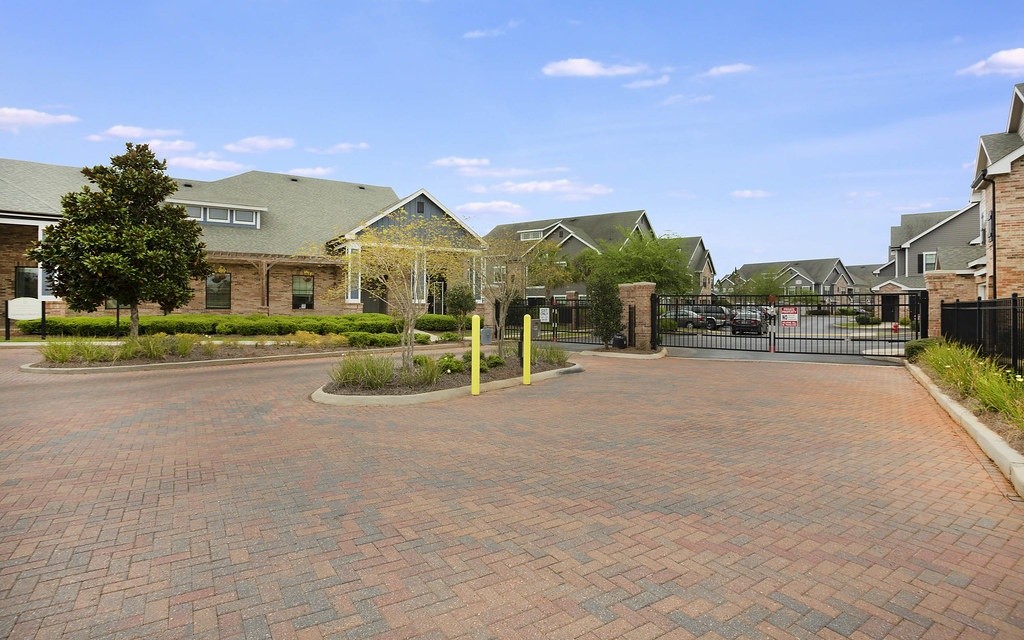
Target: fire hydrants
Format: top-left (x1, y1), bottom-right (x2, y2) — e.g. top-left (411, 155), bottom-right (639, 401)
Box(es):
top-left (891, 323), bottom-right (899, 333)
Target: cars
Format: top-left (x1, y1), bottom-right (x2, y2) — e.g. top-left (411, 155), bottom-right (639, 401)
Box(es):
top-left (725, 305), bottom-right (777, 322)
top-left (657, 309), bottom-right (707, 330)
top-left (731, 312), bottom-right (769, 335)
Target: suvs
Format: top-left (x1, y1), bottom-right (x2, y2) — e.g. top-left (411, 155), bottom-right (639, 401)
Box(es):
top-left (684, 305), bottom-right (730, 330)
